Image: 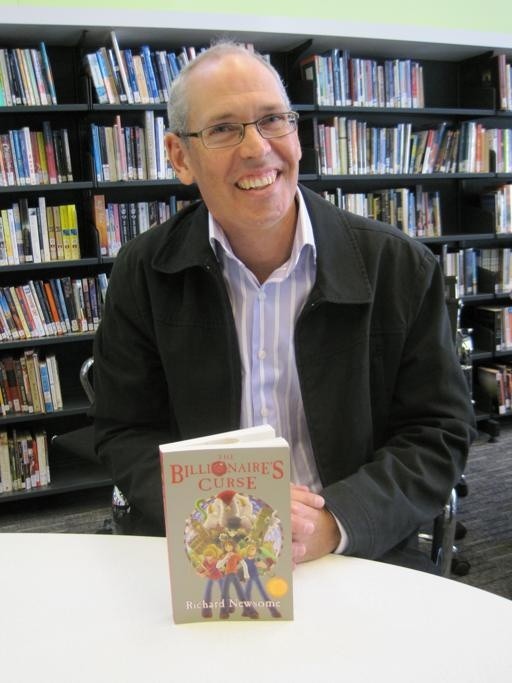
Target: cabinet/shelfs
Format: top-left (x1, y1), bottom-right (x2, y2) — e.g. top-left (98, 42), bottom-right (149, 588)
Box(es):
top-left (311, 33), bottom-right (497, 443)
top-left (0, 25), bottom-right (111, 504)
top-left (86, 28), bottom-right (311, 336)
top-left (497, 48), bottom-right (512, 420)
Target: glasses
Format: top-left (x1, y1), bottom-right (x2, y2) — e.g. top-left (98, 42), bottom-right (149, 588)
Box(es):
top-left (178, 109), bottom-right (302, 150)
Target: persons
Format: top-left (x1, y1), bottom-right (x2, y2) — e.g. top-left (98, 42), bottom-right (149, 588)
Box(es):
top-left (199, 540), bottom-right (281, 619)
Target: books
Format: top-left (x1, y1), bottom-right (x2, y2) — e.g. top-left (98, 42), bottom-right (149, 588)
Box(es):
top-left (92, 110), bottom-right (181, 184)
top-left (0, 274), bottom-right (108, 341)
top-left (299, 46), bottom-right (426, 108)
top-left (0, 196), bottom-right (81, 265)
top-left (476, 360), bottom-right (511, 414)
top-left (0, 39), bottom-right (57, 107)
top-left (158, 422), bottom-right (296, 622)
top-left (480, 182), bottom-right (511, 234)
top-left (0, 122), bottom-right (74, 185)
top-left (94, 41), bottom-right (477, 565)
top-left (322, 184), bottom-right (442, 239)
top-left (1, 432), bottom-right (51, 493)
top-left (432, 244), bottom-right (477, 297)
top-left (459, 51), bottom-right (512, 110)
top-left (300, 110), bottom-right (512, 173)
top-left (92, 194), bottom-right (192, 258)
top-left (84, 29), bottom-right (272, 104)
top-left (478, 246), bottom-right (499, 290)
top-left (0, 347), bottom-right (65, 417)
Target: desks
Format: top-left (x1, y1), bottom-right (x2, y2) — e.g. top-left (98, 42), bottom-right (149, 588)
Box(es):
top-left (0, 534), bottom-right (512, 683)
